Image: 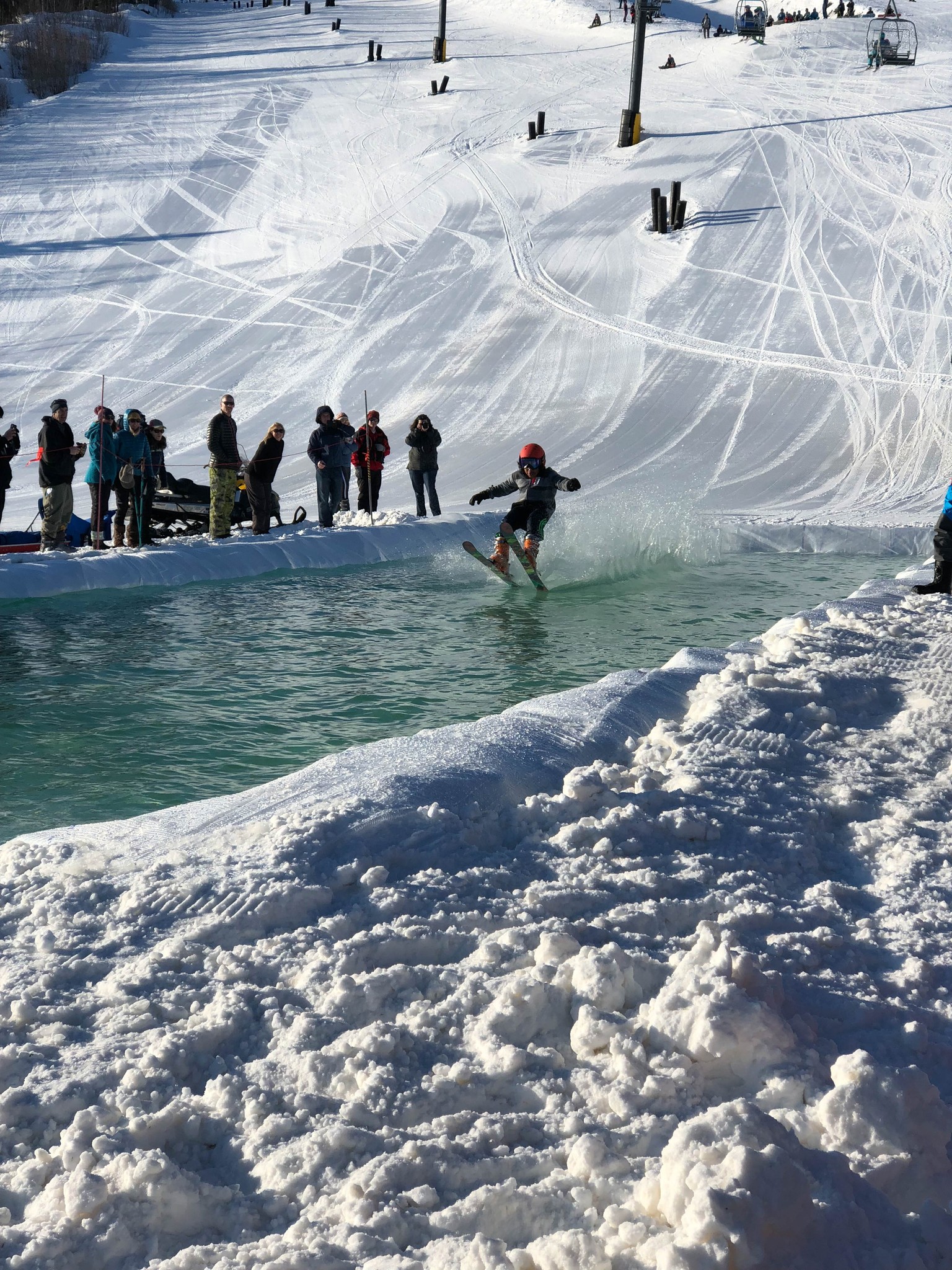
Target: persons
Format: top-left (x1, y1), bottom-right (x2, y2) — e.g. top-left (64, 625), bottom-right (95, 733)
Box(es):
top-left (591, 13), bottom-right (601, 26)
top-left (0, 407), bottom-right (16, 531)
top-left (245, 423), bottom-right (284, 536)
top-left (618, 0), bottom-right (660, 24)
top-left (702, 0), bottom-right (898, 70)
top-left (207, 395), bottom-right (243, 540)
top-left (663, 54), bottom-right (674, 67)
top-left (307, 406), bottom-right (391, 527)
top-left (470, 443), bottom-right (581, 577)
top-left (405, 415), bottom-right (442, 517)
top-left (37, 399), bottom-right (86, 553)
top-left (84, 406), bottom-right (168, 550)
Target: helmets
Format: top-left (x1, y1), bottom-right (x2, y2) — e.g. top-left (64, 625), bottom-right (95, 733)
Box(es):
top-left (516, 443), bottom-right (546, 471)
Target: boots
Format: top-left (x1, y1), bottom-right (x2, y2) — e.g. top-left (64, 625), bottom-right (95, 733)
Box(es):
top-left (911, 561), bottom-right (952, 594)
top-left (522, 533), bottom-right (540, 570)
top-left (39, 524), bottom-right (161, 552)
top-left (486, 536), bottom-right (509, 577)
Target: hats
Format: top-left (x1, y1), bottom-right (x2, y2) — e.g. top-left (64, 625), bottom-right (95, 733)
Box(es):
top-left (367, 410), bottom-right (380, 424)
top-left (336, 412), bottom-right (348, 420)
top-left (51, 399), bottom-right (167, 429)
top-left (0, 406), bottom-right (4, 419)
top-left (119, 464), bottom-right (135, 489)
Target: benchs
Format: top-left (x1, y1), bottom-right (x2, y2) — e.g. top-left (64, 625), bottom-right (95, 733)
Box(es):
top-left (151, 496), bottom-right (283, 530)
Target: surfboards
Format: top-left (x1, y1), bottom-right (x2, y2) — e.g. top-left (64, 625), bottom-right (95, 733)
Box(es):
top-left (461, 515), bottom-right (547, 592)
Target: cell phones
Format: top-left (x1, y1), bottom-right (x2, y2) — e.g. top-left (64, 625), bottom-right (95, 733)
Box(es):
top-left (326, 419), bottom-right (337, 427)
top-left (10, 424), bottom-right (17, 429)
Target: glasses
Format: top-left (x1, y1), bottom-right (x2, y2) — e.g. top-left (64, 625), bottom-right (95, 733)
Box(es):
top-left (519, 458), bottom-right (541, 469)
top-left (321, 414), bottom-right (331, 418)
top-left (271, 429), bottom-right (284, 433)
top-left (369, 418), bottom-right (378, 422)
top-left (221, 401), bottom-right (234, 405)
top-left (152, 429), bottom-right (165, 433)
top-left (103, 415), bottom-right (115, 420)
top-left (130, 419), bottom-right (141, 423)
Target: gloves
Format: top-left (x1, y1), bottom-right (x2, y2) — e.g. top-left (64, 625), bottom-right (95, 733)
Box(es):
top-left (355, 466), bottom-right (362, 479)
top-left (469, 493), bottom-right (483, 506)
top-left (567, 478), bottom-right (581, 492)
top-left (9, 424), bottom-right (21, 450)
top-left (76, 442), bottom-right (87, 457)
top-left (375, 444), bottom-right (384, 451)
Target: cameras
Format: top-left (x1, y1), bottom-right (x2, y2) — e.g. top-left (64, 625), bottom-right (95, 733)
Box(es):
top-left (421, 421), bottom-right (428, 429)
